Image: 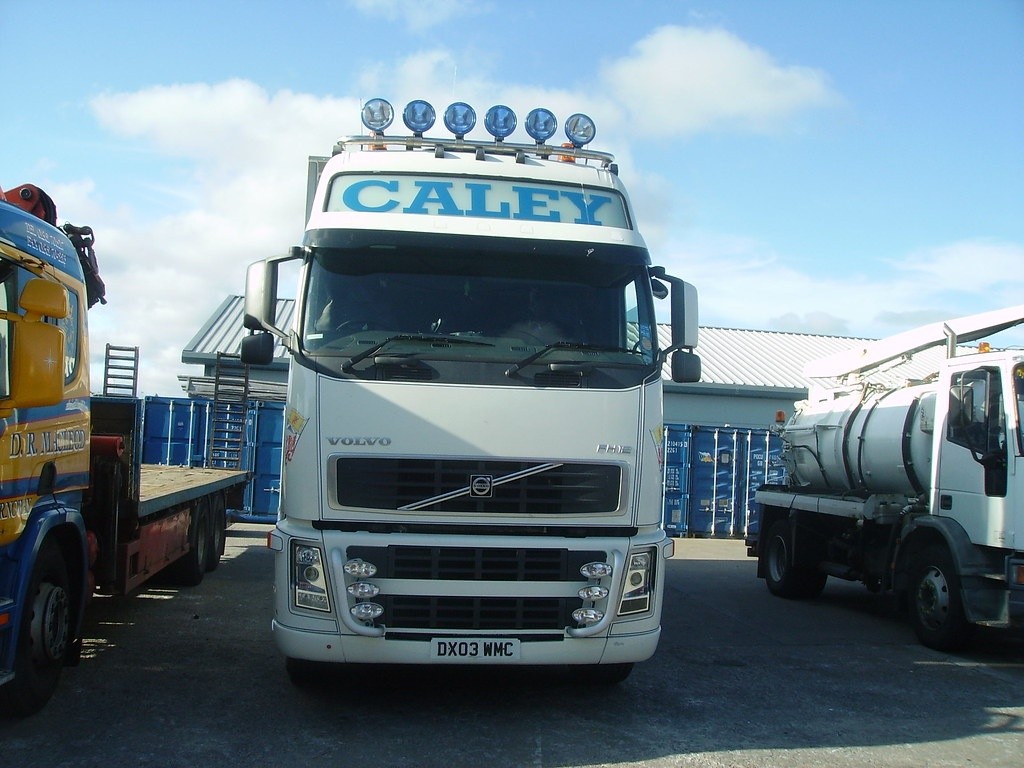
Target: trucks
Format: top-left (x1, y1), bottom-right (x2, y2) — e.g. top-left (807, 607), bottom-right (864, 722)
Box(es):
top-left (745, 303), bottom-right (1024, 652)
top-left (235, 98), bottom-right (702, 697)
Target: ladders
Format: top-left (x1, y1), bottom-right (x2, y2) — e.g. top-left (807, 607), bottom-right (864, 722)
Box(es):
top-left (208, 353), bottom-right (249, 471)
top-left (103, 345), bottom-right (138, 400)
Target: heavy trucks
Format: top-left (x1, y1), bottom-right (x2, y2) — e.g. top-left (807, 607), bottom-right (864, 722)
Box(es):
top-left (0, 184), bottom-right (255, 744)
